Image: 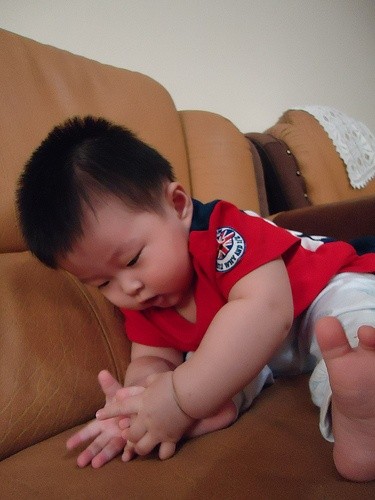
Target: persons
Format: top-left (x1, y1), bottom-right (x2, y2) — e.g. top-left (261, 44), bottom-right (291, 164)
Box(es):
top-left (14, 114), bottom-right (375, 481)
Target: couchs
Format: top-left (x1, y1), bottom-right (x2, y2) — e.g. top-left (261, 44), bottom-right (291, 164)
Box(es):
top-left (0, 28), bottom-right (375, 500)
top-left (243, 104), bottom-right (375, 208)
